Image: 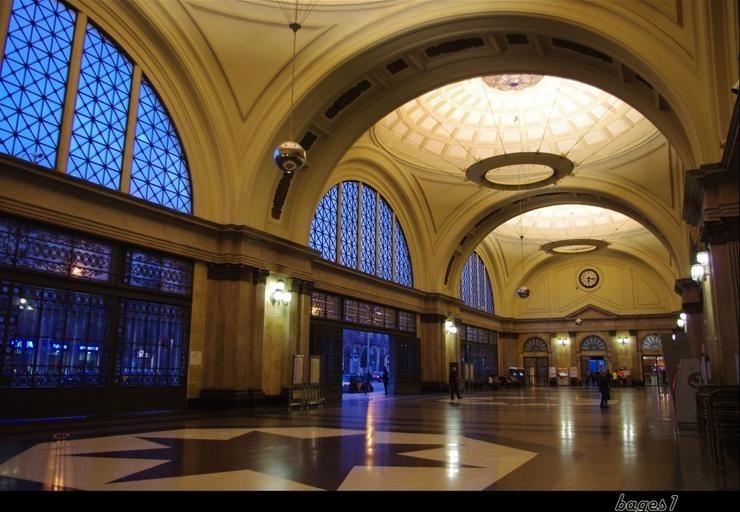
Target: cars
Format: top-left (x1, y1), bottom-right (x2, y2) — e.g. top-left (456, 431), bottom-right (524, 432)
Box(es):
top-left (342, 375), bottom-right (373, 392)
top-left (372, 374), bottom-right (382, 383)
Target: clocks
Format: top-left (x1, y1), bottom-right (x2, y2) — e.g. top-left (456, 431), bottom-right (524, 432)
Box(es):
top-left (579, 268), bottom-right (599, 288)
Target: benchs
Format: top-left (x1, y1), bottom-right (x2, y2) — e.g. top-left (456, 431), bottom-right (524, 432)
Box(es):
top-left (696, 384), bottom-right (740, 473)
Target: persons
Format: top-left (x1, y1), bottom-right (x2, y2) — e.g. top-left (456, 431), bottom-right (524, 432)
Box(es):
top-left (382, 366), bottom-right (389, 395)
top-left (582, 369), bottom-right (628, 409)
top-left (450, 367), bottom-right (462, 400)
top-left (662, 369), bottom-right (666, 384)
top-left (351, 369), bottom-right (370, 394)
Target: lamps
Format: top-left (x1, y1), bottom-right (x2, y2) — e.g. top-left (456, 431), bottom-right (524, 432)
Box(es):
top-left (446, 321), bottom-right (457, 335)
top-left (272, 279), bottom-right (292, 305)
top-left (690, 251), bottom-right (710, 284)
top-left (518, 236), bottom-right (530, 299)
top-left (558, 338), bottom-right (568, 346)
top-left (618, 336), bottom-right (629, 346)
top-left (677, 314), bottom-right (687, 334)
top-left (575, 288), bottom-right (583, 326)
top-left (274, 22), bottom-right (307, 173)
